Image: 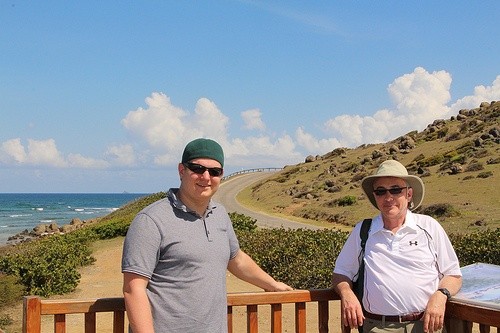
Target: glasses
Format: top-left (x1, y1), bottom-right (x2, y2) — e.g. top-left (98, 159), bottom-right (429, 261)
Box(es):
top-left (371, 186), bottom-right (412, 196)
top-left (182, 162), bottom-right (223, 176)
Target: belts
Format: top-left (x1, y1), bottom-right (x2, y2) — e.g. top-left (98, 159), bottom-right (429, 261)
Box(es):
top-left (363, 310), bottom-right (424, 322)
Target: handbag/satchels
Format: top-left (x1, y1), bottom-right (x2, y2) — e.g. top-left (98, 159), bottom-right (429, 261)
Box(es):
top-left (352, 218), bottom-right (372, 302)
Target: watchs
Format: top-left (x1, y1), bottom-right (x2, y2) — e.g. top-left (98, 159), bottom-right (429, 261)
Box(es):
top-left (437, 288), bottom-right (451, 299)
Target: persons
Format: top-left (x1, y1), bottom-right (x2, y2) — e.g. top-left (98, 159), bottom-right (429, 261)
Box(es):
top-left (121, 139), bottom-right (296, 333)
top-left (332, 160), bottom-right (462, 333)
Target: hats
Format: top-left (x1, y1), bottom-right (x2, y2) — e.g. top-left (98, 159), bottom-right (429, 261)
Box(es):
top-left (361, 160), bottom-right (424, 211)
top-left (182, 138), bottom-right (224, 168)
